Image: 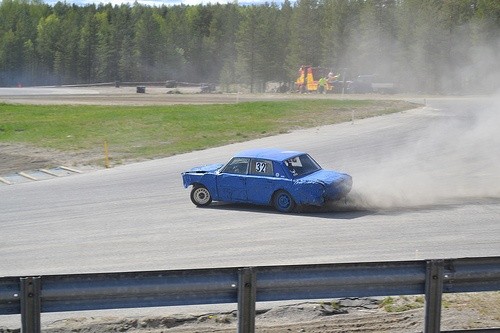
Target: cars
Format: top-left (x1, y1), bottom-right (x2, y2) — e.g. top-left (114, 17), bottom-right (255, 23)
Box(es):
top-left (181, 150), bottom-right (352, 213)
